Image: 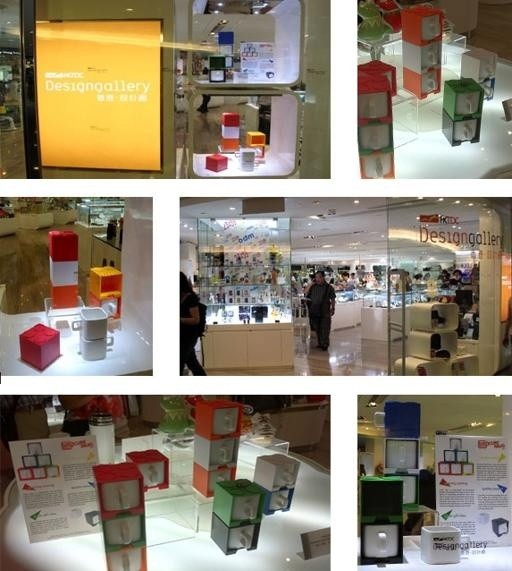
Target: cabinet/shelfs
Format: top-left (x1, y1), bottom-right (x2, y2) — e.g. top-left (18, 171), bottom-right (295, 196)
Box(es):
top-left (195, 216), bottom-right (430, 370)
top-left (73, 203), bottom-right (125, 272)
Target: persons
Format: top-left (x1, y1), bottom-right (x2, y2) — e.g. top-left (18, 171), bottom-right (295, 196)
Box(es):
top-left (180, 271), bottom-right (208, 376)
top-left (405, 264), bottom-right (479, 293)
top-left (57, 396), bottom-right (130, 442)
top-left (0, 200), bottom-right (15, 219)
top-left (292, 268), bottom-right (380, 298)
top-left (0, 396), bottom-right (53, 453)
top-left (305, 270), bottom-right (336, 353)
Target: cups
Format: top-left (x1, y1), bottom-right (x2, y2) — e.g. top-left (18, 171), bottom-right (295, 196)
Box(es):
top-left (422, 523), bottom-right (471, 563)
top-left (235, 148), bottom-right (255, 162)
top-left (89, 397), bottom-right (301, 571)
top-left (72, 298), bottom-right (118, 361)
top-left (363, 525), bottom-right (396, 560)
top-left (359, 15), bottom-right (496, 178)
top-left (371, 401), bottom-right (417, 506)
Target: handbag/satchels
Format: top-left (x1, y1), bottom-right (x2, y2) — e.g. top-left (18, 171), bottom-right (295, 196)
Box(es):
top-left (196, 301), bottom-right (208, 335)
